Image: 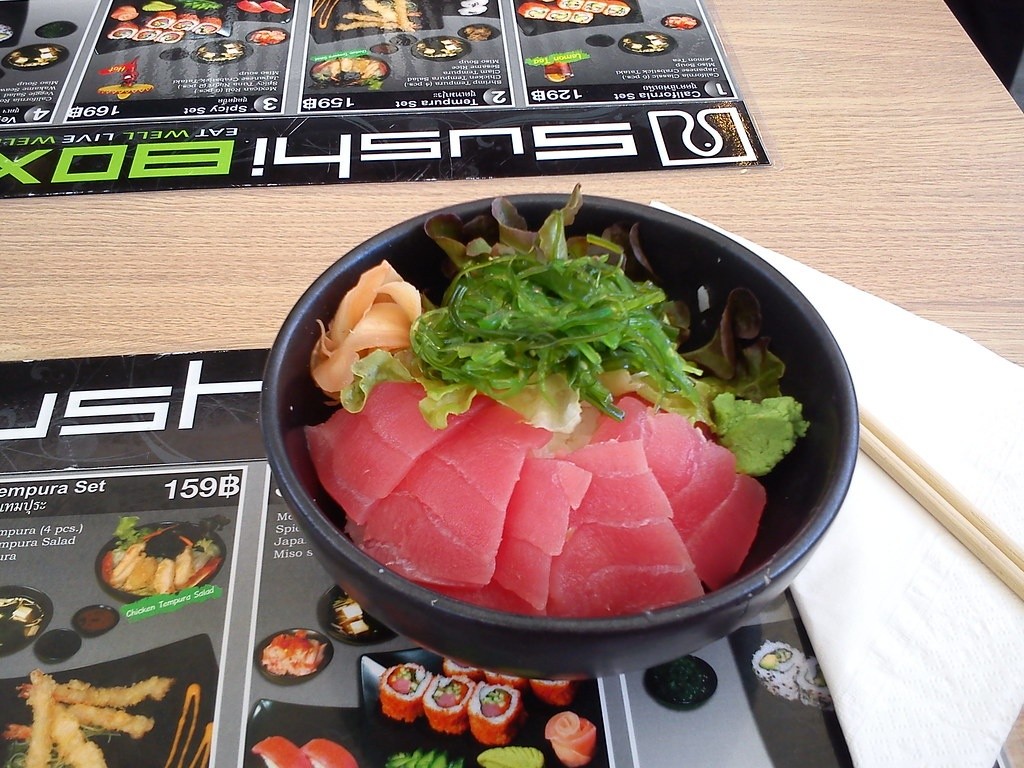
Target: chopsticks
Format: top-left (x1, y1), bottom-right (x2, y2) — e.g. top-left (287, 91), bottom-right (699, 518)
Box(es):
top-left (856, 404), bottom-right (1024, 602)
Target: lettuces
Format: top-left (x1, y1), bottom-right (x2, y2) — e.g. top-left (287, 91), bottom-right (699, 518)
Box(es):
top-left (337, 178), bottom-right (788, 435)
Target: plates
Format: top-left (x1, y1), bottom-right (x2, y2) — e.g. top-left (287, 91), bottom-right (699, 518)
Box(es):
top-left (95, 0), bottom-right (295, 54)
top-left (411, 35), bottom-right (472, 62)
top-left (359, 646), bottom-right (604, 752)
top-left (1, 43), bottom-right (69, 71)
top-left (514, 1), bottom-right (644, 36)
top-left (243, 698), bottom-right (610, 768)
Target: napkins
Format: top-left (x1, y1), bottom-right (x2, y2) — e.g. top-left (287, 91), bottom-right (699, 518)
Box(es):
top-left (649, 198), bottom-right (1024, 768)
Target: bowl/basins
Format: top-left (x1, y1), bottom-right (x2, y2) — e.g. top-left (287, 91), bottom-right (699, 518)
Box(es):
top-left (258, 193), bottom-right (861, 681)
top-left (660, 12), bottom-right (702, 30)
top-left (618, 30), bottom-right (677, 57)
top-left (0, 585), bottom-right (54, 659)
top-left (245, 27), bottom-right (290, 46)
top-left (34, 627), bottom-right (82, 664)
top-left (316, 583), bottom-right (400, 646)
top-left (72, 604), bottom-right (120, 638)
top-left (35, 20), bottom-right (77, 39)
top-left (457, 23), bottom-right (501, 42)
top-left (190, 39), bottom-right (254, 66)
top-left (253, 627), bottom-right (334, 686)
top-left (94, 522), bottom-right (227, 606)
top-left (310, 54), bottom-right (391, 86)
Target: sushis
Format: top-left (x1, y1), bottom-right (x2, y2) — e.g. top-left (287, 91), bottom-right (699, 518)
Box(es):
top-left (252, 651), bottom-right (581, 768)
top-left (751, 640), bottom-right (835, 712)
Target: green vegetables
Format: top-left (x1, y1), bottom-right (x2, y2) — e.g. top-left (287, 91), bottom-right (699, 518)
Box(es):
top-left (0, 723), bottom-right (122, 768)
top-left (112, 514), bottom-right (231, 553)
